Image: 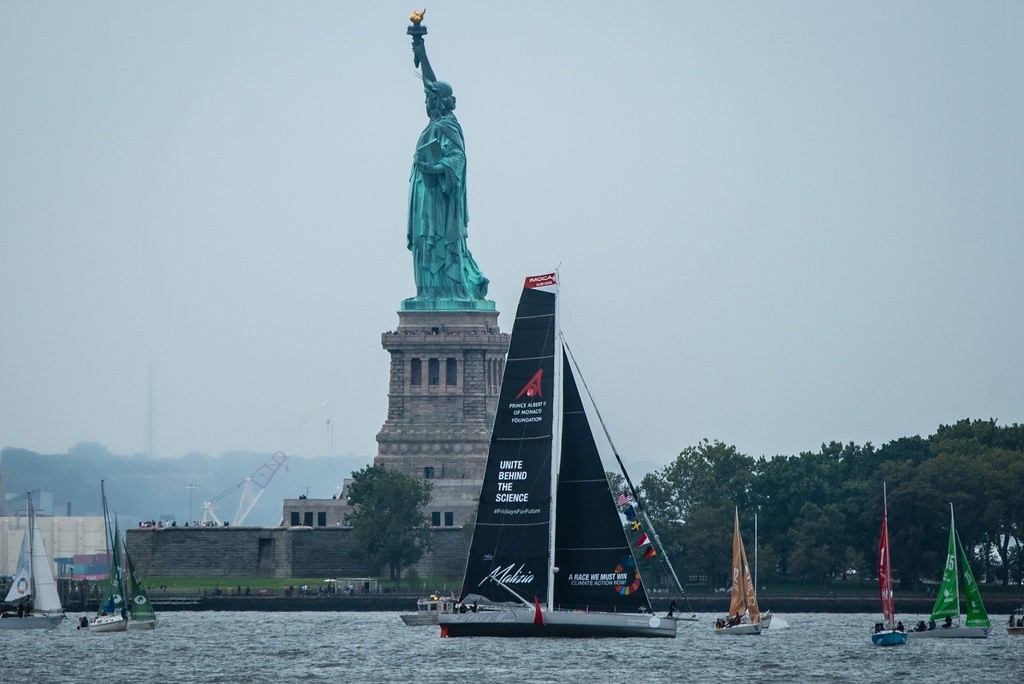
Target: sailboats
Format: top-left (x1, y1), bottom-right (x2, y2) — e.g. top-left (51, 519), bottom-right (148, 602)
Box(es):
top-left (712, 505), bottom-right (774, 634)
top-left (76, 478), bottom-right (161, 631)
top-left (868, 480), bottom-right (911, 647)
top-left (0, 493), bottom-right (67, 629)
top-left (437, 262), bottom-right (704, 643)
top-left (905, 503), bottom-right (998, 640)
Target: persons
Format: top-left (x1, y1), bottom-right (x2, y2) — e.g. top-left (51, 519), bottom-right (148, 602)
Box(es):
top-left (299, 493), bottom-right (307, 500)
top-left (139, 519), bottom-right (230, 528)
top-left (335, 518), bottom-right (350, 526)
top-left (1009, 614), bottom-right (1024, 628)
top-left (386, 328), bottom-right (511, 336)
top-left (152, 583), bottom-right (168, 591)
top-left (101, 607), bottom-right (128, 620)
top-left (435, 590), bottom-right (478, 613)
top-left (715, 612), bottom-right (740, 629)
top-left (284, 582), bottom-right (354, 598)
top-left (72, 577), bottom-right (98, 600)
top-left (79, 615), bottom-right (88, 628)
top-left (874, 621), bottom-right (904, 634)
top-left (331, 492), bottom-right (353, 502)
top-left (279, 520), bottom-right (307, 526)
top-left (0, 600), bottom-right (33, 618)
top-left (910, 614), bottom-right (952, 632)
top-left (202, 584), bottom-right (274, 597)
top-left (667, 598), bottom-right (676, 617)
top-left (407, 9), bottom-right (490, 301)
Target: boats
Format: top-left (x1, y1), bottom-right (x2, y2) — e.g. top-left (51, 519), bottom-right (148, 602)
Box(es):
top-left (399, 594), bottom-right (459, 625)
top-left (1004, 608), bottom-right (1024, 635)
